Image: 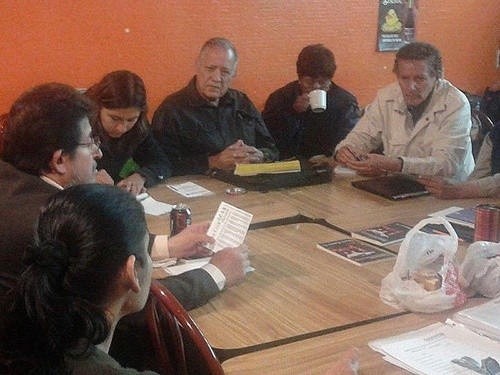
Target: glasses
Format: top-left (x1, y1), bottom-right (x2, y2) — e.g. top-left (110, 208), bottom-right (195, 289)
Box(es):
top-left (78, 136), bottom-right (101, 154)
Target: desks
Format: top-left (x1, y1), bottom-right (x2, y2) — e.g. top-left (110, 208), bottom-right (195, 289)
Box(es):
top-left (128, 154), bottom-right (500, 375)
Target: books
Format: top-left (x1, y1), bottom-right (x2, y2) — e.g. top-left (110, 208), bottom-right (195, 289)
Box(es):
top-left (317, 237), bottom-right (397, 267)
top-left (350, 220), bottom-right (417, 247)
top-left (350, 174), bottom-right (430, 201)
top-left (419, 201), bottom-right (500, 243)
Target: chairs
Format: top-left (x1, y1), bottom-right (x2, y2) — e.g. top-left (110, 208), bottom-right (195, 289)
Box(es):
top-left (144, 277), bottom-right (224, 375)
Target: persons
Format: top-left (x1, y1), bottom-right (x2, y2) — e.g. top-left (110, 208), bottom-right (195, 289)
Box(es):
top-left (262, 43), bottom-right (361, 159)
top-left (416, 89), bottom-right (500, 199)
top-left (0, 81), bottom-right (251, 313)
top-left (150, 36), bottom-right (278, 176)
top-left (332, 42), bottom-right (476, 185)
top-left (1, 182), bottom-right (155, 375)
top-left (77, 70), bottom-right (172, 197)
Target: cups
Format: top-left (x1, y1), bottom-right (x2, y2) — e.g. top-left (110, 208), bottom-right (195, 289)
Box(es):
top-left (305, 90), bottom-right (327, 112)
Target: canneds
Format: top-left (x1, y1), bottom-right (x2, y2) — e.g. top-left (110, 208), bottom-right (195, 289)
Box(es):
top-left (473, 203), bottom-right (500, 242)
top-left (169, 204), bottom-right (192, 237)
